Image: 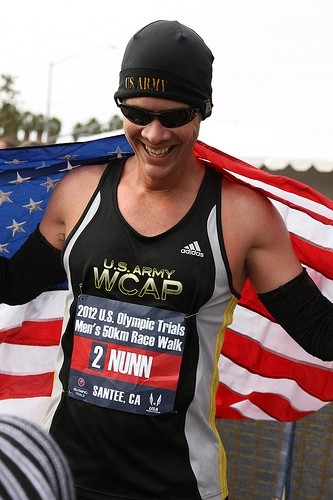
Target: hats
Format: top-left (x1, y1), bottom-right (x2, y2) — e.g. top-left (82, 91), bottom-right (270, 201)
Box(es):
top-left (113, 20), bottom-right (214, 120)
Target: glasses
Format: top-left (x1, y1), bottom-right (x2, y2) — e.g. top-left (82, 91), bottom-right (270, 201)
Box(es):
top-left (114, 97), bottom-right (200, 128)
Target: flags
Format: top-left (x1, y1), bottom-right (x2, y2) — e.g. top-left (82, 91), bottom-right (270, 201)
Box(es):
top-left (1, 131), bottom-right (333, 429)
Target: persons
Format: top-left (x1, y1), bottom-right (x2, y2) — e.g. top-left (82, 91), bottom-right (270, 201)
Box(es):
top-left (1, 20), bottom-right (333, 500)
